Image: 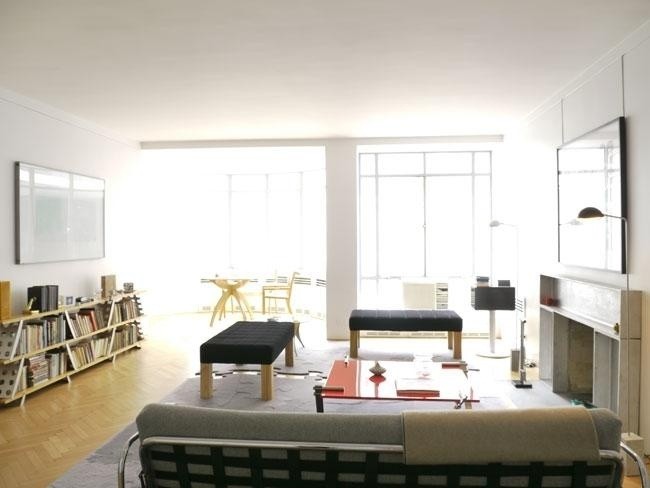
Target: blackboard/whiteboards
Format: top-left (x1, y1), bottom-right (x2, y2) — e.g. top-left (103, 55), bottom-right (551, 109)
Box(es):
top-left (557, 115), bottom-right (627, 275)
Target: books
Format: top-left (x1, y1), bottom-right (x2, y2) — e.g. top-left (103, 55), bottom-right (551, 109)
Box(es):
top-left (0, 276), bottom-right (136, 394)
top-left (396, 378), bottom-right (440, 395)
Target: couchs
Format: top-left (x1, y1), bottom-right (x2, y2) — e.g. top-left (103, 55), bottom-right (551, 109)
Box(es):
top-left (117, 403), bottom-right (650, 488)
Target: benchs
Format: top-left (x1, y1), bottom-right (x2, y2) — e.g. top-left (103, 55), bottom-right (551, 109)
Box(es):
top-left (349, 310), bottom-right (462, 359)
top-left (200, 321), bottom-right (295, 400)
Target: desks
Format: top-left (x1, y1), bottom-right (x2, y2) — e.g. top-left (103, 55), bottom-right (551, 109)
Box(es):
top-left (471, 287), bottom-right (515, 359)
top-left (209, 279), bottom-right (251, 326)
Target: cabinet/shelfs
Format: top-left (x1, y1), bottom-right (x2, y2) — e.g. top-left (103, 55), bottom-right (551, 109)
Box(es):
top-left (0, 290), bottom-right (145, 406)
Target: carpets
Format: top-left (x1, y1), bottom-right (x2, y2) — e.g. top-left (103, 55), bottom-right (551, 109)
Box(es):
top-left (48, 351), bottom-right (571, 487)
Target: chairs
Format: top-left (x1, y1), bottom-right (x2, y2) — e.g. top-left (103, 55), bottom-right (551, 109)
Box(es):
top-left (262, 272), bottom-right (295, 315)
top-left (268, 318), bottom-right (304, 356)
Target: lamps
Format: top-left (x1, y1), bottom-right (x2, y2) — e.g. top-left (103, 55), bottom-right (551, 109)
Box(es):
top-left (578, 207), bottom-right (644, 477)
